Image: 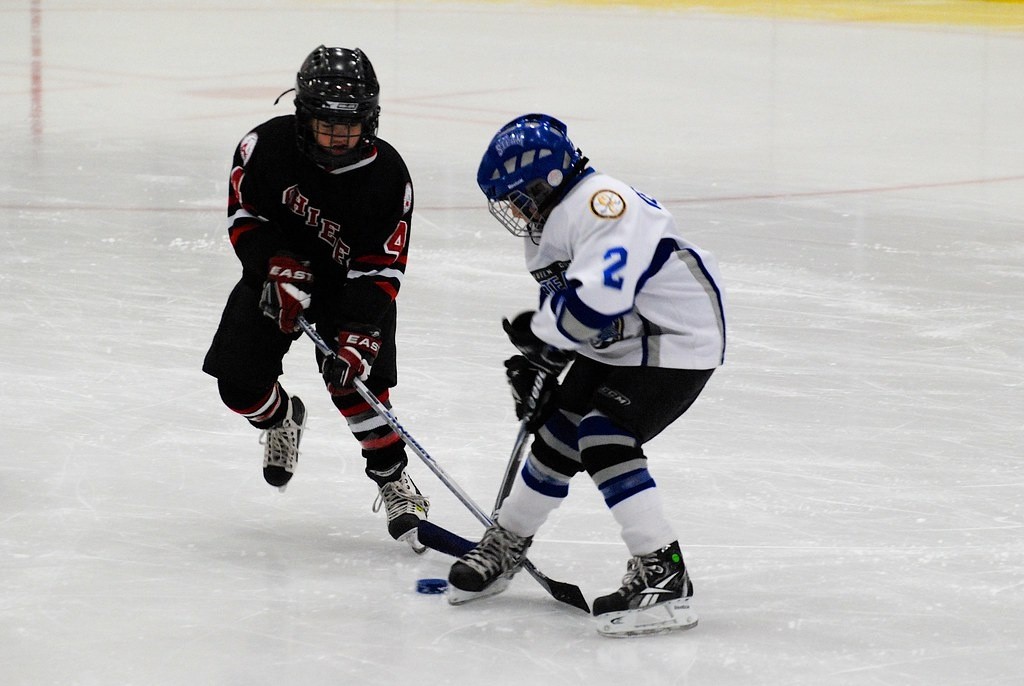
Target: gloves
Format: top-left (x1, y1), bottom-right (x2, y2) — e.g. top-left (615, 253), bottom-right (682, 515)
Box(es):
top-left (503, 310), bottom-right (577, 432)
top-left (268, 256), bottom-right (310, 332)
top-left (322, 317), bottom-right (381, 397)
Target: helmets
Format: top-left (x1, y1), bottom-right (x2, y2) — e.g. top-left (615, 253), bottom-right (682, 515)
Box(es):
top-left (295, 46), bottom-right (379, 132)
top-left (478, 114), bottom-right (595, 200)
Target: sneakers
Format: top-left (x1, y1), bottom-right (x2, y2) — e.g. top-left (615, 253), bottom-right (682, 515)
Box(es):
top-left (592, 540), bottom-right (698, 629)
top-left (366, 452), bottom-right (430, 554)
top-left (263, 396), bottom-right (305, 488)
top-left (450, 507), bottom-right (536, 605)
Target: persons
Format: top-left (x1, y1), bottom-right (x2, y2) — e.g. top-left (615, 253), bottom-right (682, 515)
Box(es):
top-left (447, 113), bottom-right (726, 638)
top-left (202, 44), bottom-right (429, 555)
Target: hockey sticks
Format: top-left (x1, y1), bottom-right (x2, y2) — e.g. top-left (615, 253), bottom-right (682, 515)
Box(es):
top-left (414, 365), bottom-right (555, 559)
top-left (295, 313), bottom-right (593, 615)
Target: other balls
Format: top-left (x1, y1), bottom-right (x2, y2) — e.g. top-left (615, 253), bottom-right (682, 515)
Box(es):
top-left (412, 577), bottom-right (446, 596)
